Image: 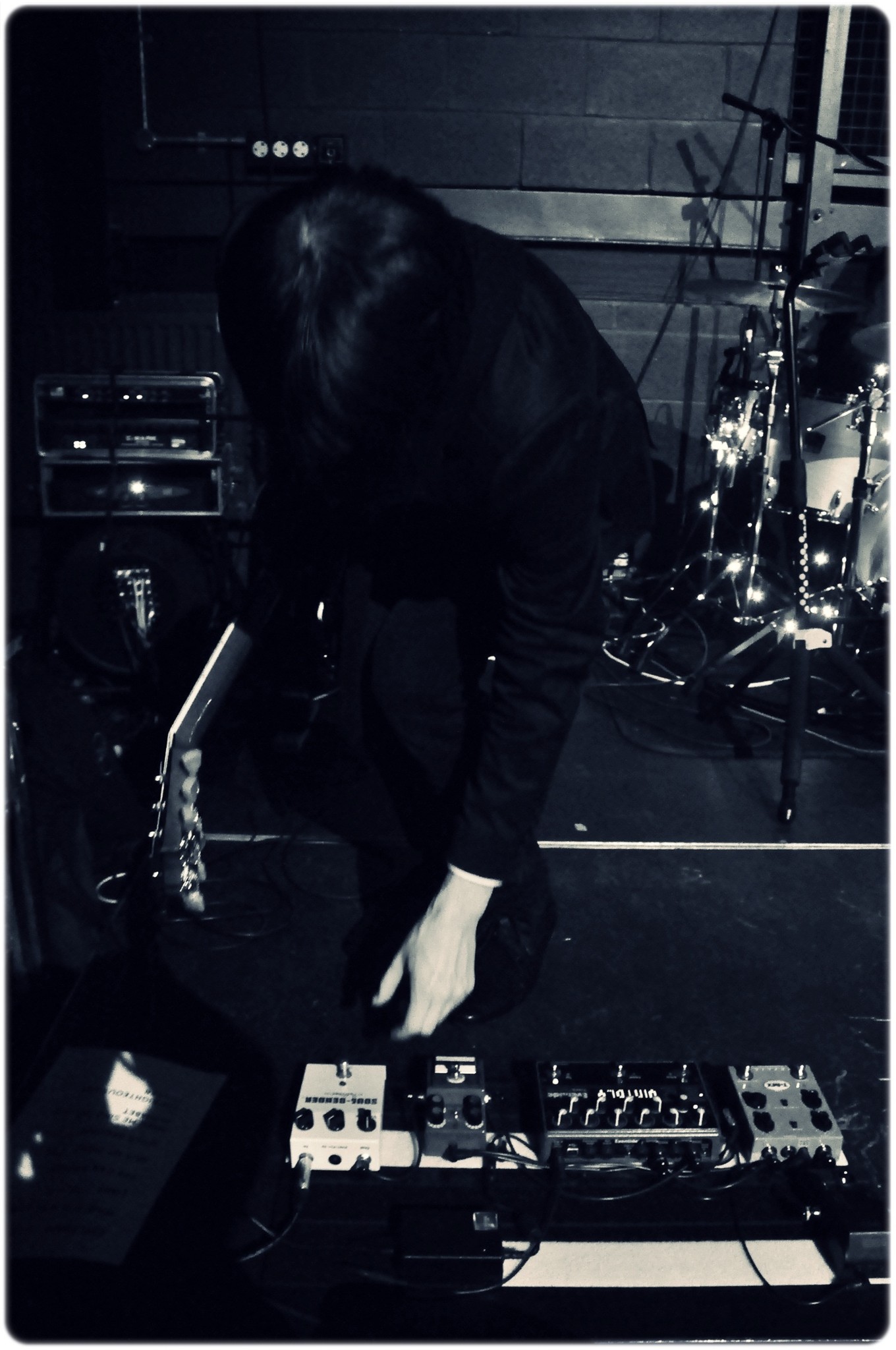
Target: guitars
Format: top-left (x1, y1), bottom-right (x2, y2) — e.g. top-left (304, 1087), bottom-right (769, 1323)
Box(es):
top-left (141, 477), bottom-right (315, 918)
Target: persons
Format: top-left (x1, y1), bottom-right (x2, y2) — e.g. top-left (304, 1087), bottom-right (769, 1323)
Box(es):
top-left (223, 169), bottom-right (654, 1075)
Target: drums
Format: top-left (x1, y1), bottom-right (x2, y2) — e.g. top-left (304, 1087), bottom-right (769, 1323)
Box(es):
top-left (765, 380), bottom-right (890, 531)
top-left (712, 376), bottom-right (767, 493)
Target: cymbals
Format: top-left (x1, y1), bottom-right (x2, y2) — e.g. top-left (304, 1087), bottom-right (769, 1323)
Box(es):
top-left (680, 271), bottom-right (869, 317)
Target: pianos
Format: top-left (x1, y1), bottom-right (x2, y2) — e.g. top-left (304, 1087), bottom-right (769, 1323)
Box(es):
top-left (412, 174), bottom-right (795, 260)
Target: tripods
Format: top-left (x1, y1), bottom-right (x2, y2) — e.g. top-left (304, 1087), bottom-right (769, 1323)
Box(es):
top-left (611, 231), bottom-right (891, 833)
